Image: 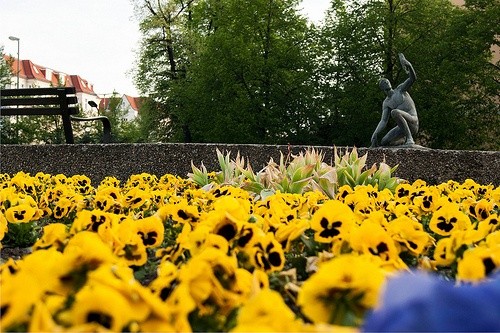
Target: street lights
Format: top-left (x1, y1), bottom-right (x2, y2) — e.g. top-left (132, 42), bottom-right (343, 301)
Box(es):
top-left (9, 35), bottom-right (21, 144)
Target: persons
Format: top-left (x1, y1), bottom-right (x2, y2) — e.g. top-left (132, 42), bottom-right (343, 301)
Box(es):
top-left (369, 52), bottom-right (420, 148)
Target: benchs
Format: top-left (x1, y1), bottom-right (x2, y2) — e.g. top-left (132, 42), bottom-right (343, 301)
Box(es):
top-left (0, 87), bottom-right (111, 144)
